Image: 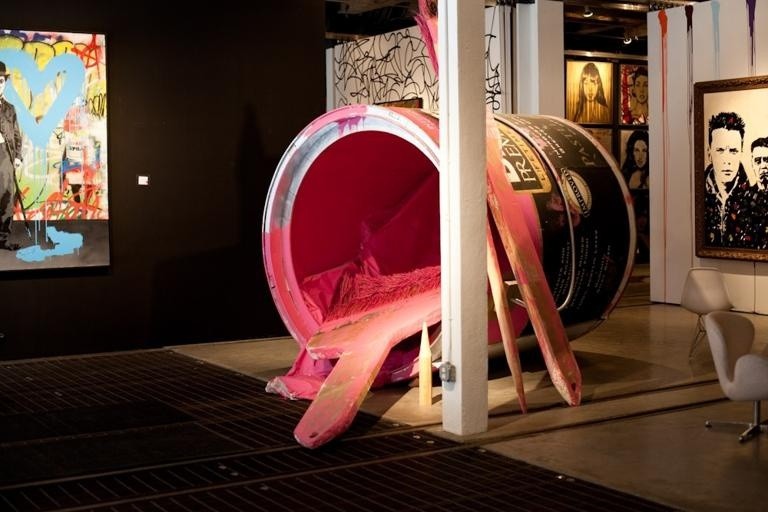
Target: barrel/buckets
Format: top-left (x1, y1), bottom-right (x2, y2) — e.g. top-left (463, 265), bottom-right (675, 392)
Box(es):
top-left (261, 102), bottom-right (638, 391)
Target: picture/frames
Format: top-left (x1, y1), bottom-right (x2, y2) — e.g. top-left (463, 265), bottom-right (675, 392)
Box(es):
top-left (564, 54), bottom-right (650, 195)
top-left (694, 76), bottom-right (768, 262)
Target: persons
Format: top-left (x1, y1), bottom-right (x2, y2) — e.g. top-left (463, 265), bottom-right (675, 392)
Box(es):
top-left (0, 63), bottom-right (26, 250)
top-left (737, 137), bottom-right (768, 251)
top-left (570, 59), bottom-right (611, 128)
top-left (700, 108), bottom-right (753, 254)
top-left (616, 130), bottom-right (649, 195)
top-left (621, 65), bottom-right (649, 124)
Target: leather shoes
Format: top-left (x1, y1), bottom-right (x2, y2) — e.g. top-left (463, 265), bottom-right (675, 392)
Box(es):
top-left (1, 236), bottom-right (20, 251)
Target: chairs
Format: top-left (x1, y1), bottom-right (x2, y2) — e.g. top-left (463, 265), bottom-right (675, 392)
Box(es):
top-left (702, 310), bottom-right (767, 446)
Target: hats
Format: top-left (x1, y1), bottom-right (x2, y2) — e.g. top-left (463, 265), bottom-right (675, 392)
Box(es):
top-left (0, 60), bottom-right (11, 78)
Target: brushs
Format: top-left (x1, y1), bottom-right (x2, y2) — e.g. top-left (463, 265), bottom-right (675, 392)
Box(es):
top-left (413, 0), bottom-right (583, 416)
top-left (292, 264), bottom-right (441, 452)
